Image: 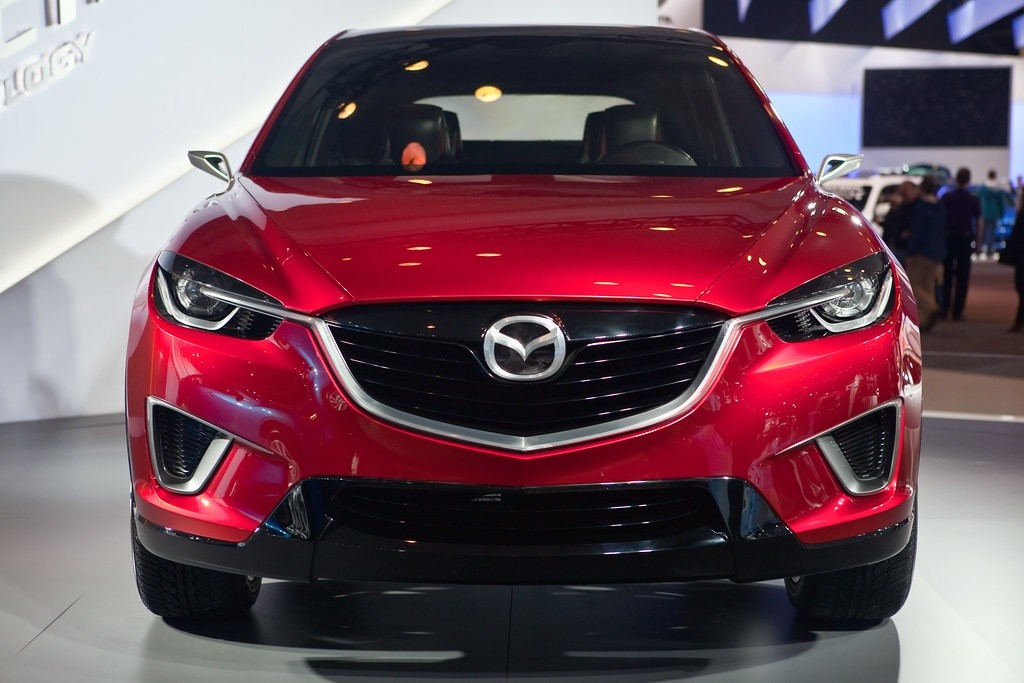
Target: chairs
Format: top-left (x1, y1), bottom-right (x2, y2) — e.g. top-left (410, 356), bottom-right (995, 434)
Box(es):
top-left (385, 103), bottom-right (449, 174)
top-left (596, 101), bottom-right (698, 166)
top-left (579, 108), bottom-right (603, 166)
top-left (445, 110), bottom-right (463, 155)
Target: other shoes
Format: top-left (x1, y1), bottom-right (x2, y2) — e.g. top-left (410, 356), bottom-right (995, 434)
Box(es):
top-left (920, 309), bottom-right (941, 332)
top-left (1006, 325), bottom-right (1024, 333)
top-left (954, 317), bottom-right (966, 322)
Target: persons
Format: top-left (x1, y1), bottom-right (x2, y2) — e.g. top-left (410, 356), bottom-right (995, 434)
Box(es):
top-left (900, 181), bottom-right (948, 330)
top-left (936, 168), bottom-right (985, 321)
top-left (1004, 182), bottom-right (1024, 332)
top-left (975, 168), bottom-right (1006, 262)
top-left (874, 179), bottom-right (920, 267)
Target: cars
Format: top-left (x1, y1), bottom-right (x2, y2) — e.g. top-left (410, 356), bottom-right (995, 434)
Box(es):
top-left (824, 173), bottom-right (925, 237)
top-left (127, 25), bottom-right (922, 629)
